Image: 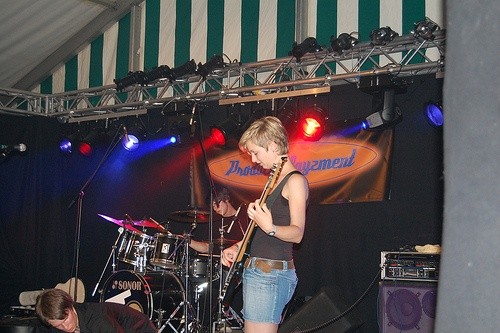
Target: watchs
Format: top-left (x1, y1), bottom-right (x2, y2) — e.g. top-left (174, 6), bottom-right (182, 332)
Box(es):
top-left (267, 225), bottom-right (276, 237)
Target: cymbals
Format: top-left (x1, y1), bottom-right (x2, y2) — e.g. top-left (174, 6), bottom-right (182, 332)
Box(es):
top-left (96, 213), bottom-right (141, 233)
top-left (168, 210), bottom-right (220, 223)
top-left (118, 218), bottom-right (160, 228)
top-left (202, 237), bottom-right (237, 244)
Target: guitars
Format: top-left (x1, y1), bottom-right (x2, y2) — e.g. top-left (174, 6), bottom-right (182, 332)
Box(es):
top-left (219, 156), bottom-right (288, 309)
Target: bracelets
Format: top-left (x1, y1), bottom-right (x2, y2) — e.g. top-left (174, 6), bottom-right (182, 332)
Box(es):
top-left (234, 244), bottom-right (239, 247)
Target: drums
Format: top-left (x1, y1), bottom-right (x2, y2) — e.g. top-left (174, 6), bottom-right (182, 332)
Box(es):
top-left (149, 232), bottom-right (187, 269)
top-left (116, 228), bottom-right (158, 268)
top-left (184, 258), bottom-right (213, 282)
top-left (100, 269), bottom-right (186, 333)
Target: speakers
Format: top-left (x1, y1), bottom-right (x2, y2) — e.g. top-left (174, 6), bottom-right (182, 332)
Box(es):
top-left (277, 287), bottom-right (366, 333)
top-left (379, 280), bottom-right (438, 333)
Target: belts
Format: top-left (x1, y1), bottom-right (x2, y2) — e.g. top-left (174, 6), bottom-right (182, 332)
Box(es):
top-left (243, 257), bottom-right (294, 273)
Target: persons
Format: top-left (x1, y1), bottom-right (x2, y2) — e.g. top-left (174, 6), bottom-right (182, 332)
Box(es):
top-left (178, 187), bottom-right (249, 271)
top-left (220, 116), bottom-right (310, 333)
top-left (35, 289), bottom-right (158, 333)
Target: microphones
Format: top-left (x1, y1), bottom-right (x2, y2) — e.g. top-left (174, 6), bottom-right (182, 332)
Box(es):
top-left (125, 214), bottom-right (134, 227)
top-left (190, 103), bottom-right (196, 125)
top-left (122, 124), bottom-right (129, 142)
top-left (0, 144), bottom-right (27, 152)
top-left (227, 204), bottom-right (243, 233)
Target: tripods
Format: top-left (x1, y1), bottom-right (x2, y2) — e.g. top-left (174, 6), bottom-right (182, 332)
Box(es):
top-left (158, 221), bottom-right (244, 333)
